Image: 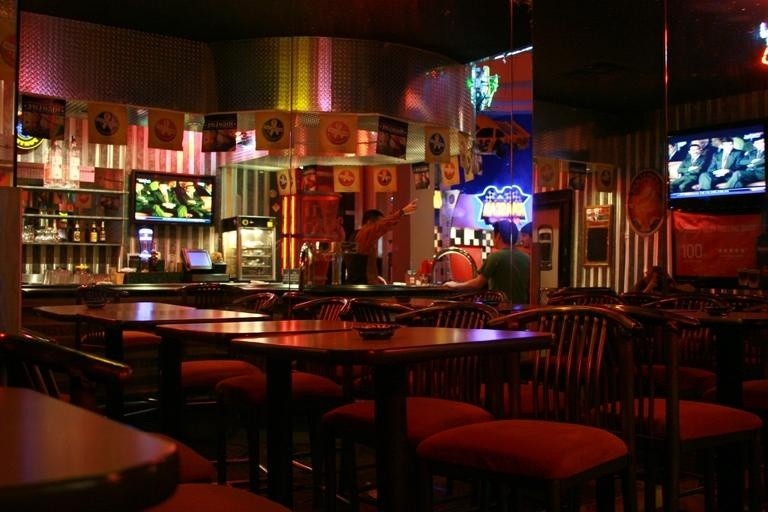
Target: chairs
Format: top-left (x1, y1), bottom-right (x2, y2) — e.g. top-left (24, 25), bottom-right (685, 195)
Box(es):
top-left (0, 247), bottom-right (767, 511)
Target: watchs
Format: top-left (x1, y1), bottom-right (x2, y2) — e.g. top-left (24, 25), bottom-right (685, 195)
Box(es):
top-left (399, 208), bottom-right (406, 218)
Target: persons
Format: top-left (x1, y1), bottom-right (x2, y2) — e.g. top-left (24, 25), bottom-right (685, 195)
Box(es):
top-left (443, 220), bottom-right (530, 305)
top-left (515, 224), bottom-right (532, 255)
top-left (135, 179), bottom-right (212, 219)
top-left (355, 198), bottom-right (420, 286)
top-left (669, 136), bottom-right (766, 191)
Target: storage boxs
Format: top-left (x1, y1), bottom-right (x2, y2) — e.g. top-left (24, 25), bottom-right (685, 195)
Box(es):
top-left (15, 161), bottom-right (45, 185)
top-left (78, 166), bottom-right (125, 191)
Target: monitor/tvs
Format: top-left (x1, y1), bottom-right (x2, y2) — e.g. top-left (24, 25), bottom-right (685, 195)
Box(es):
top-left (665, 124), bottom-right (768, 212)
top-left (129, 169), bottom-right (215, 226)
top-left (181, 248), bottom-right (212, 270)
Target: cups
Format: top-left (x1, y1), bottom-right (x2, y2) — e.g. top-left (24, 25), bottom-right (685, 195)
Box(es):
top-left (421, 276), bottom-right (429, 286)
top-left (24, 224), bottom-right (57, 243)
top-left (176, 262), bottom-right (183, 273)
top-left (42, 270), bottom-right (75, 285)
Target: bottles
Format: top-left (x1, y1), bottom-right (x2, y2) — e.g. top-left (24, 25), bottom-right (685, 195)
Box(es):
top-left (331, 252), bottom-right (342, 286)
top-left (405, 269), bottom-right (418, 285)
top-left (90, 220), bottom-right (98, 242)
top-left (73, 219), bottom-right (81, 241)
top-left (66, 137), bottom-right (81, 189)
top-left (299, 243), bottom-right (315, 289)
top-left (169, 260), bottom-right (175, 273)
top-left (99, 219), bottom-right (106, 242)
top-left (45, 139), bottom-right (64, 187)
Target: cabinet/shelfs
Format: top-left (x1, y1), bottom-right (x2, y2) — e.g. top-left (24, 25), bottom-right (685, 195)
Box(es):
top-left (19, 185), bottom-right (129, 246)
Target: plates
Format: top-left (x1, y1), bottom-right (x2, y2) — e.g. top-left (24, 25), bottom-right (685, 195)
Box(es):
top-left (16, 111), bottom-right (43, 150)
top-left (350, 324), bottom-right (400, 338)
